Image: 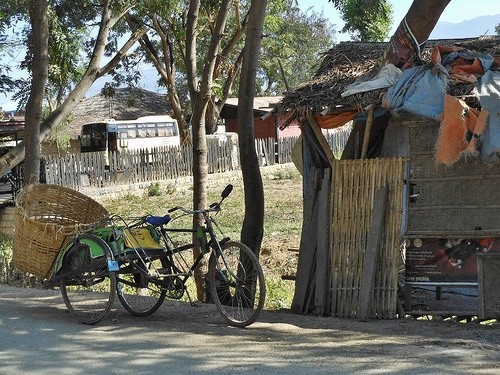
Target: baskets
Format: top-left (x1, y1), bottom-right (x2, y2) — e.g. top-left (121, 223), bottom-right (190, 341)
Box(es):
top-left (10, 183), bottom-right (110, 280)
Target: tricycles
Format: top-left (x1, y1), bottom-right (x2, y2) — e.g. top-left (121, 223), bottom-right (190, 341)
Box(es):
top-left (39, 183), bottom-right (266, 327)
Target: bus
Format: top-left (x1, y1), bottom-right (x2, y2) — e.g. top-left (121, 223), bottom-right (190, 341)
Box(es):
top-left (78, 115), bottom-right (181, 173)
